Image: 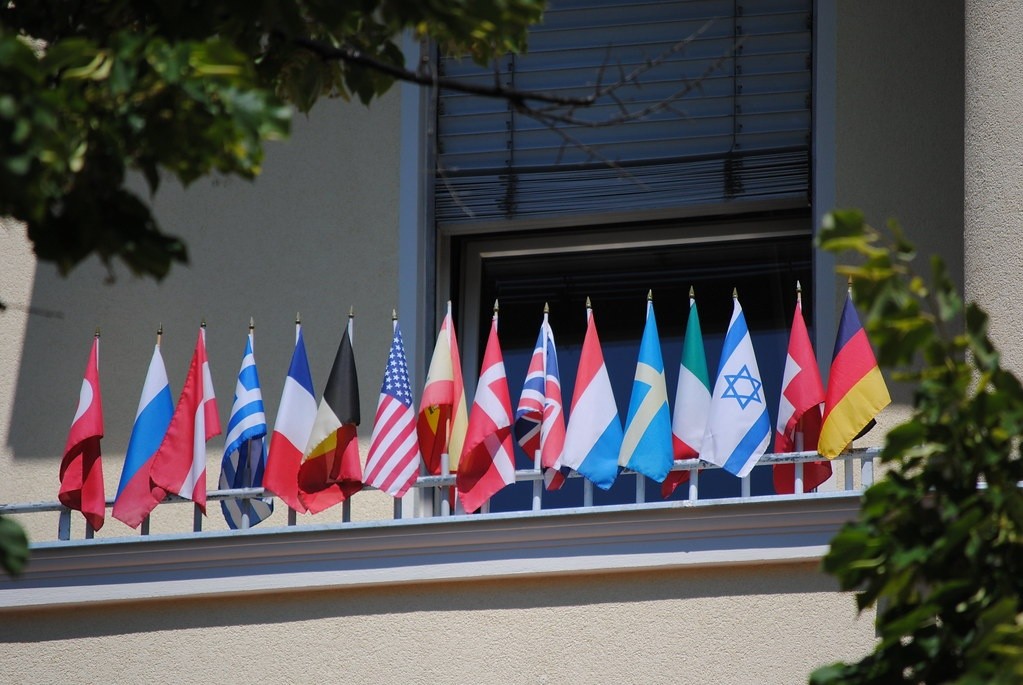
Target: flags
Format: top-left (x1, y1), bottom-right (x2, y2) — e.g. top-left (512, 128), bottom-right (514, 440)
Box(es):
top-left (698, 300), bottom-right (771, 478)
top-left (149, 330), bottom-right (221, 517)
top-left (456, 319), bottom-right (515, 513)
top-left (514, 322), bottom-right (566, 491)
top-left (661, 300), bottom-right (712, 499)
top-left (559, 313), bottom-right (624, 490)
top-left (773, 301), bottom-right (833, 495)
top-left (263, 329), bottom-right (318, 515)
top-left (417, 315), bottom-right (468, 510)
top-left (817, 293), bottom-right (891, 460)
top-left (58, 342), bottom-right (105, 531)
top-left (618, 302), bottom-right (674, 483)
top-left (218, 336), bottom-right (274, 531)
top-left (362, 322), bottom-right (421, 498)
top-left (296, 325), bottom-right (363, 515)
top-left (112, 348), bottom-right (175, 529)
